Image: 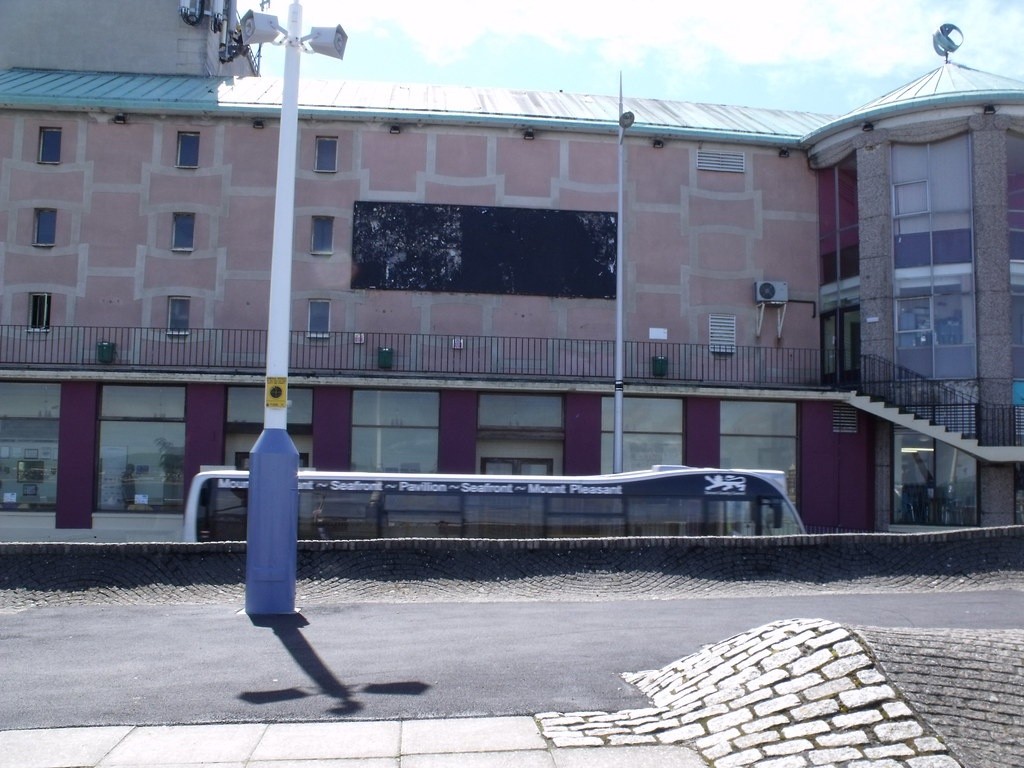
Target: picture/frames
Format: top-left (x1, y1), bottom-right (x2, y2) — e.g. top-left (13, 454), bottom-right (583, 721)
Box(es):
top-left (24, 448), bottom-right (38, 459)
top-left (23, 484), bottom-right (37, 496)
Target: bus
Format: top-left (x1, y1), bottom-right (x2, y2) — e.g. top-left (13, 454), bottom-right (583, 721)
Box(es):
top-left (189, 464), bottom-right (804, 541)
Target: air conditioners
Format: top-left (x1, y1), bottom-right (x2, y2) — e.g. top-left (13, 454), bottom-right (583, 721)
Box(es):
top-left (754, 281), bottom-right (788, 303)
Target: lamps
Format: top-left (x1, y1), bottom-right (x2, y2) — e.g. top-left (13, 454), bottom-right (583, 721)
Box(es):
top-left (252, 120), bottom-right (264, 128)
top-left (779, 149), bottom-right (790, 157)
top-left (984, 105), bottom-right (995, 115)
top-left (654, 140), bottom-right (663, 148)
top-left (862, 122), bottom-right (874, 131)
top-left (390, 125), bottom-right (400, 134)
top-left (524, 131), bottom-right (534, 140)
top-left (113, 114), bottom-right (126, 124)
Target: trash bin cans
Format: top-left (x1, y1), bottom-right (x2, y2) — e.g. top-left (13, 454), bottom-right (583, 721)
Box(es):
top-left (377, 347), bottom-right (393, 369)
top-left (96, 341), bottom-right (115, 364)
top-left (653, 356), bottom-right (668, 377)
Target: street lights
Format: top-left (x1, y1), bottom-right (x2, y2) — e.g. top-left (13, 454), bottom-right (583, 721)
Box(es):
top-left (613, 111), bottom-right (635, 473)
